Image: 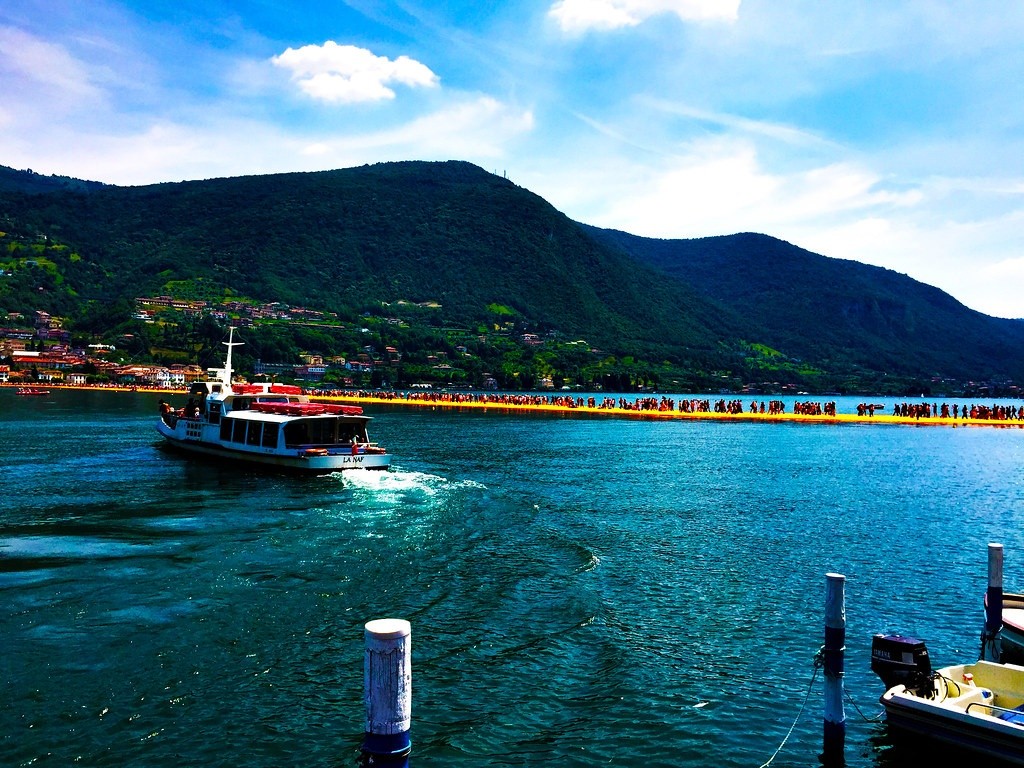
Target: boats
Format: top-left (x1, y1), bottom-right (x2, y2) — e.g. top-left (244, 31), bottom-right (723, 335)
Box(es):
top-left (983, 590), bottom-right (1024, 655)
top-left (869, 631), bottom-right (1024, 767)
top-left (155, 377), bottom-right (393, 476)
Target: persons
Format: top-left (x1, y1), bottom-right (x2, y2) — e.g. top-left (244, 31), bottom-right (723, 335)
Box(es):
top-left (824, 401), bottom-right (836, 417)
top-left (159, 400), bottom-right (171, 414)
top-left (598, 396), bottom-right (615, 409)
top-left (970, 404), bottom-right (1024, 421)
top-left (953, 404), bottom-right (958, 419)
top-left (679, 399), bottom-right (711, 413)
top-left (793, 400), bottom-right (822, 415)
top-left (869, 403), bottom-right (874, 417)
top-left (857, 403), bottom-right (866, 416)
top-left (27, 388), bottom-right (31, 393)
top-left (184, 398), bottom-right (196, 418)
top-left (767, 400), bottom-right (785, 415)
top-left (940, 402), bottom-right (950, 418)
top-left (962, 405), bottom-right (968, 419)
top-left (750, 400), bottom-right (758, 413)
top-left (760, 402), bottom-right (765, 413)
top-left (932, 403), bottom-right (938, 417)
top-left (302, 388), bottom-right (595, 409)
top-left (619, 396), bottom-right (674, 411)
top-left (18, 387), bottom-right (23, 392)
top-left (714, 399), bottom-right (743, 414)
top-left (893, 402), bottom-right (931, 420)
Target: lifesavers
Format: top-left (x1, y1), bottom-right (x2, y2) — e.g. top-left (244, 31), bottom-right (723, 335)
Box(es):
top-left (306, 448), bottom-right (327, 457)
